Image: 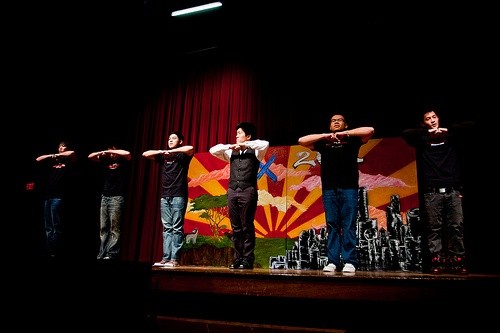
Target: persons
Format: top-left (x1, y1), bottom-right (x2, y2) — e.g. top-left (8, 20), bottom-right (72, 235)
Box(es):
top-left (36, 140), bottom-right (77, 255)
top-left (298, 114), bottom-right (375, 273)
top-left (87, 143), bottom-right (133, 261)
top-left (142, 131), bottom-right (193, 267)
top-left (209, 122), bottom-right (269, 269)
top-left (404, 106), bottom-right (480, 276)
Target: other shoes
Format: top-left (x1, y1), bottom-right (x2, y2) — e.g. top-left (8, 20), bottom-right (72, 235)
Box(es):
top-left (452, 256), bottom-right (469, 274)
top-left (431, 258), bottom-right (440, 273)
top-left (343, 263), bottom-right (355, 272)
top-left (154, 260), bottom-right (168, 266)
top-left (238, 263), bottom-right (253, 269)
top-left (230, 261), bottom-right (242, 269)
top-left (165, 259), bottom-right (180, 267)
top-left (323, 263), bottom-right (336, 271)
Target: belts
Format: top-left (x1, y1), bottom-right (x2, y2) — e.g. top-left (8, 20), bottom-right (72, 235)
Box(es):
top-left (426, 188), bottom-right (462, 193)
